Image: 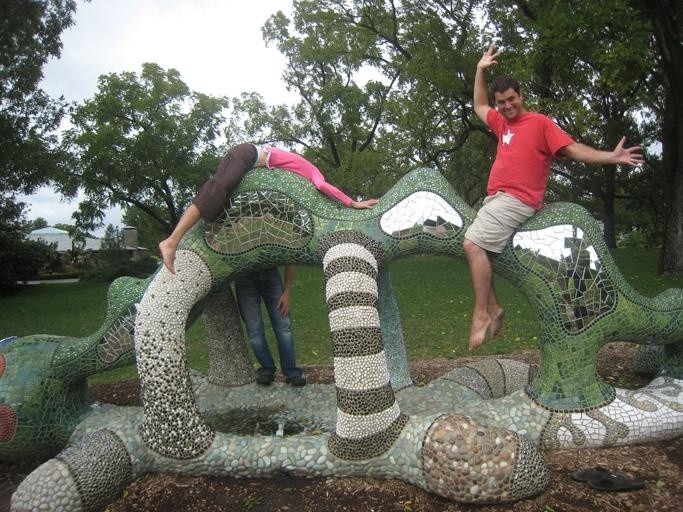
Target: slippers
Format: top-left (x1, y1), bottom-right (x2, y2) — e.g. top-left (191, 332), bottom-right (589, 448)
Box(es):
top-left (571, 467), bottom-right (647, 492)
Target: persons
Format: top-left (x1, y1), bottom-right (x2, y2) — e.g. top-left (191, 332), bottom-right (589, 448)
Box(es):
top-left (236, 266), bottom-right (306, 386)
top-left (463, 44), bottom-right (645, 351)
top-left (159, 145), bottom-right (379, 274)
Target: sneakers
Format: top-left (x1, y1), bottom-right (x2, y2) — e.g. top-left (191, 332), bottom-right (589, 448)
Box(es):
top-left (290, 376), bottom-right (306, 386)
top-left (260, 375), bottom-right (271, 385)
